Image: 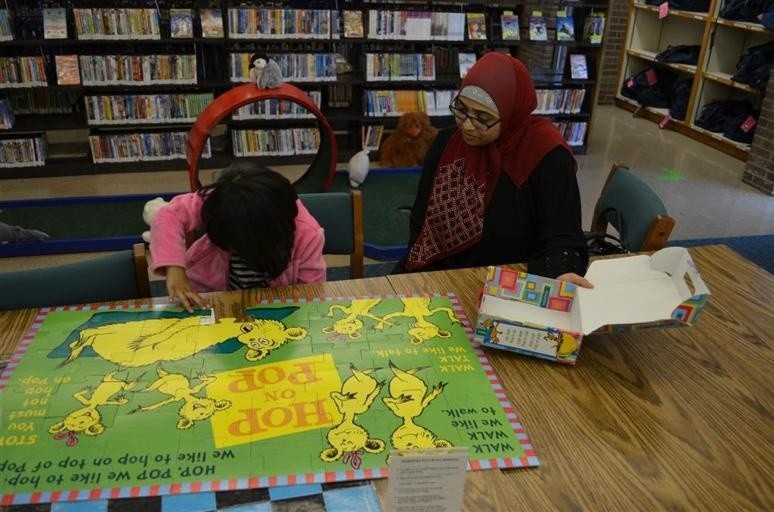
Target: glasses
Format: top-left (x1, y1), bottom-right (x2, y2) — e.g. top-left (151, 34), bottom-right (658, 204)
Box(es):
top-left (449, 94), bottom-right (500, 132)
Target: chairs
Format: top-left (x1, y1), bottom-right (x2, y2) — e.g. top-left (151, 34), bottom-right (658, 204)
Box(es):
top-left (185, 188), bottom-right (365, 279)
top-left (0, 241), bottom-right (152, 304)
top-left (581, 161), bottom-right (677, 260)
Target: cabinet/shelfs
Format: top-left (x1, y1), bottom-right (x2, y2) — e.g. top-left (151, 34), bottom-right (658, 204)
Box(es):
top-left (0, 2), bottom-right (611, 181)
top-left (613, 2), bottom-right (773, 165)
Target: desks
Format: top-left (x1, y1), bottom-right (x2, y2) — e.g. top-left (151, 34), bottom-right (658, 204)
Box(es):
top-left (0, 243), bottom-right (772, 512)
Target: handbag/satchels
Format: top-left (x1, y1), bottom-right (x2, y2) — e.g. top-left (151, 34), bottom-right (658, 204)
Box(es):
top-left (536, 207), bottom-right (633, 279)
top-left (620, 1), bottom-right (772, 141)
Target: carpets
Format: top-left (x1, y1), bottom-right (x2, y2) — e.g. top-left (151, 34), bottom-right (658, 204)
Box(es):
top-left (326, 233), bottom-right (774, 283)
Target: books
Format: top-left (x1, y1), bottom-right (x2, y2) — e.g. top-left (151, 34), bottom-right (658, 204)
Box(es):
top-left (328, 10), bottom-right (364, 107)
top-left (229, 9), bottom-right (341, 156)
top-left (361, 10), bottom-right (605, 146)
top-left (80, 55), bottom-right (214, 163)
top-left (42, 8), bottom-right (67, 40)
top-left (1, 11), bottom-right (46, 169)
top-left (74, 6), bottom-right (225, 38)
top-left (56, 55), bottom-right (81, 85)
top-left (45, 88), bottom-right (81, 113)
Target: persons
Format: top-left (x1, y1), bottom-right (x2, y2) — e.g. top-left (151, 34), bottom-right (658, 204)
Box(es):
top-left (149, 163), bottom-right (327, 314)
top-left (403, 52), bottom-right (594, 288)
